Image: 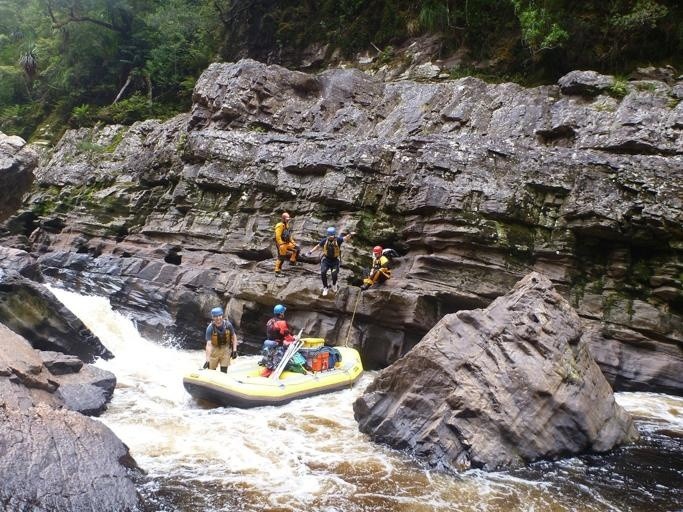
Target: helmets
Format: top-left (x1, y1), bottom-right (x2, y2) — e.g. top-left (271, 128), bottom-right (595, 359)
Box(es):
top-left (210, 307), bottom-right (224, 318)
top-left (274, 304), bottom-right (287, 314)
top-left (373, 246), bottom-right (383, 254)
top-left (282, 213), bottom-right (291, 220)
top-left (327, 227), bottom-right (336, 235)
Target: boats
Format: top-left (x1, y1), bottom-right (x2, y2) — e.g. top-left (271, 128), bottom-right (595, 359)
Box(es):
top-left (183, 346), bottom-right (363, 407)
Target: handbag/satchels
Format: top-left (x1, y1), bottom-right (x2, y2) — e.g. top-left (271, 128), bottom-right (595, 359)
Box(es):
top-left (317, 346), bottom-right (342, 369)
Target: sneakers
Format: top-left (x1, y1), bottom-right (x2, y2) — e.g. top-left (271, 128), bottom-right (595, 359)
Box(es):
top-left (331, 281), bottom-right (338, 292)
top-left (275, 272), bottom-right (285, 277)
top-left (360, 284), bottom-right (368, 290)
top-left (323, 287), bottom-right (330, 296)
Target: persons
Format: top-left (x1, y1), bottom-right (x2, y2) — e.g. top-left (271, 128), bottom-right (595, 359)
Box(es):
top-left (306, 226), bottom-right (352, 297)
top-left (202, 306), bottom-right (237, 373)
top-left (272, 212), bottom-right (299, 277)
top-left (266, 304), bottom-right (291, 348)
top-left (359, 245), bottom-right (399, 291)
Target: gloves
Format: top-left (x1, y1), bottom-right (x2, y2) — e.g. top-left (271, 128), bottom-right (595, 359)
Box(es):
top-left (230, 351), bottom-right (237, 359)
top-left (203, 361), bottom-right (209, 369)
